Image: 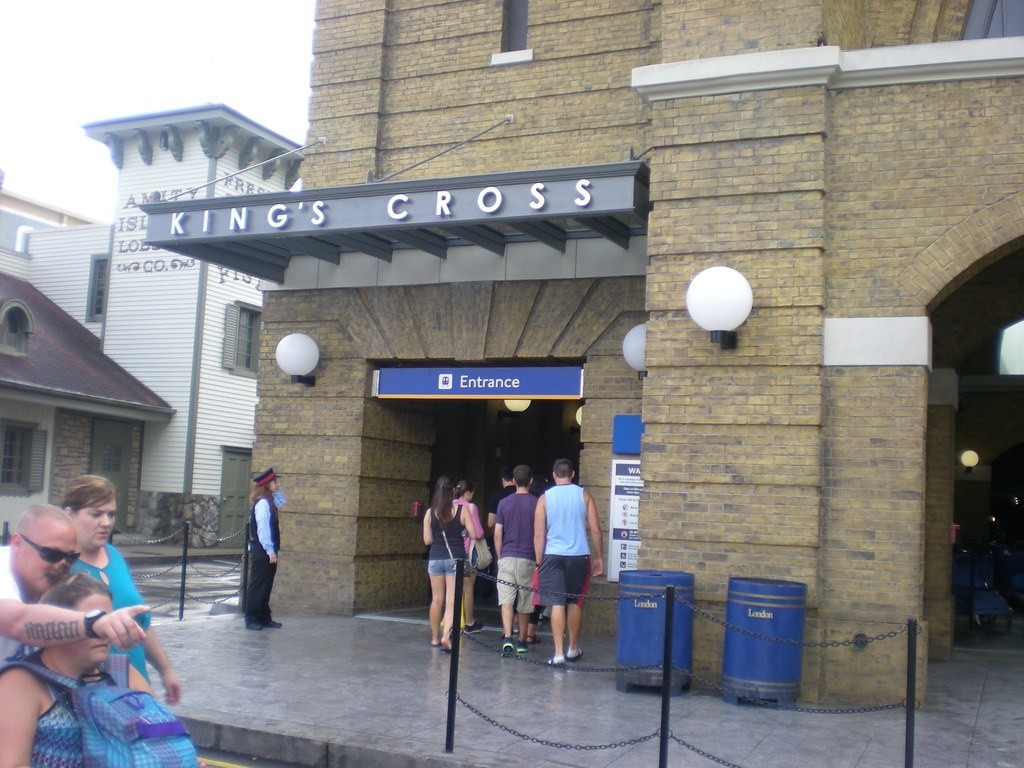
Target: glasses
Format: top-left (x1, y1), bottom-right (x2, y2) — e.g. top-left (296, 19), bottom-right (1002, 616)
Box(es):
top-left (21, 534), bottom-right (81, 563)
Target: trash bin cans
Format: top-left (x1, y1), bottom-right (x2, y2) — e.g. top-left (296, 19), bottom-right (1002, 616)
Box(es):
top-left (725, 576), bottom-right (808, 710)
top-left (616, 569), bottom-right (695, 698)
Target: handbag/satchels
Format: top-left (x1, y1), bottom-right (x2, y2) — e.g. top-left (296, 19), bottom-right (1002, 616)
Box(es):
top-left (469, 537), bottom-right (493, 570)
top-left (453, 560), bottom-right (471, 577)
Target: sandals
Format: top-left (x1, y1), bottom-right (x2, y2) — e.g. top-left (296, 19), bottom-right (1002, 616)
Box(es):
top-left (527, 634), bottom-right (541, 644)
top-left (500, 630), bottom-right (514, 639)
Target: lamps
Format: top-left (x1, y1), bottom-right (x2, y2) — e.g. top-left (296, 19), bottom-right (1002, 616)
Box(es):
top-left (624, 323), bottom-right (648, 377)
top-left (498, 399), bottom-right (583, 428)
top-left (276, 334), bottom-right (321, 386)
top-left (962, 451), bottom-right (979, 473)
top-left (686, 266), bottom-right (754, 350)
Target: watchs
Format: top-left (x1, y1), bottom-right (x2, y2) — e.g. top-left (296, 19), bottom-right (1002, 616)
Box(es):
top-left (84, 610), bottom-right (106, 640)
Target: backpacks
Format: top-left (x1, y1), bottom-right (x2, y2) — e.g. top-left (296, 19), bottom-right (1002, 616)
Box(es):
top-left (7, 653), bottom-right (198, 768)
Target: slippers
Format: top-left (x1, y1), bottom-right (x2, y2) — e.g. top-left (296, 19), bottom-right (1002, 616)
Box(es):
top-left (547, 658), bottom-right (565, 667)
top-left (431, 641), bottom-right (441, 647)
top-left (566, 649), bottom-right (583, 662)
top-left (440, 647), bottom-right (452, 653)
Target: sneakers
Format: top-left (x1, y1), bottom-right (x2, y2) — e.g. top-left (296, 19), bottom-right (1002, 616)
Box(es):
top-left (517, 640), bottom-right (528, 653)
top-left (463, 620), bottom-right (483, 634)
top-left (501, 639), bottom-right (515, 658)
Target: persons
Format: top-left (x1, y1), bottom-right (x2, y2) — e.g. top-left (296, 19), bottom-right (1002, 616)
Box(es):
top-left (532, 459), bottom-right (605, 666)
top-left (0, 503), bottom-right (152, 669)
top-left (488, 466), bottom-right (543, 655)
top-left (60, 474), bottom-right (184, 704)
top-left (422, 475), bottom-right (476, 653)
top-left (0, 572), bottom-right (206, 768)
top-left (245, 467), bottom-right (283, 630)
top-left (452, 479), bottom-right (485, 634)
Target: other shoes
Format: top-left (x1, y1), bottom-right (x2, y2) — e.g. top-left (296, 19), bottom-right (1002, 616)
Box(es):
top-left (513, 625), bottom-right (519, 633)
top-left (264, 621), bottom-right (282, 628)
top-left (543, 608), bottom-right (551, 617)
top-left (539, 613), bottom-right (543, 620)
top-left (246, 622), bottom-right (262, 630)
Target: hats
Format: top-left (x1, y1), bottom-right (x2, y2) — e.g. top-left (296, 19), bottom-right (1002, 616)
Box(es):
top-left (254, 468), bottom-right (282, 486)
